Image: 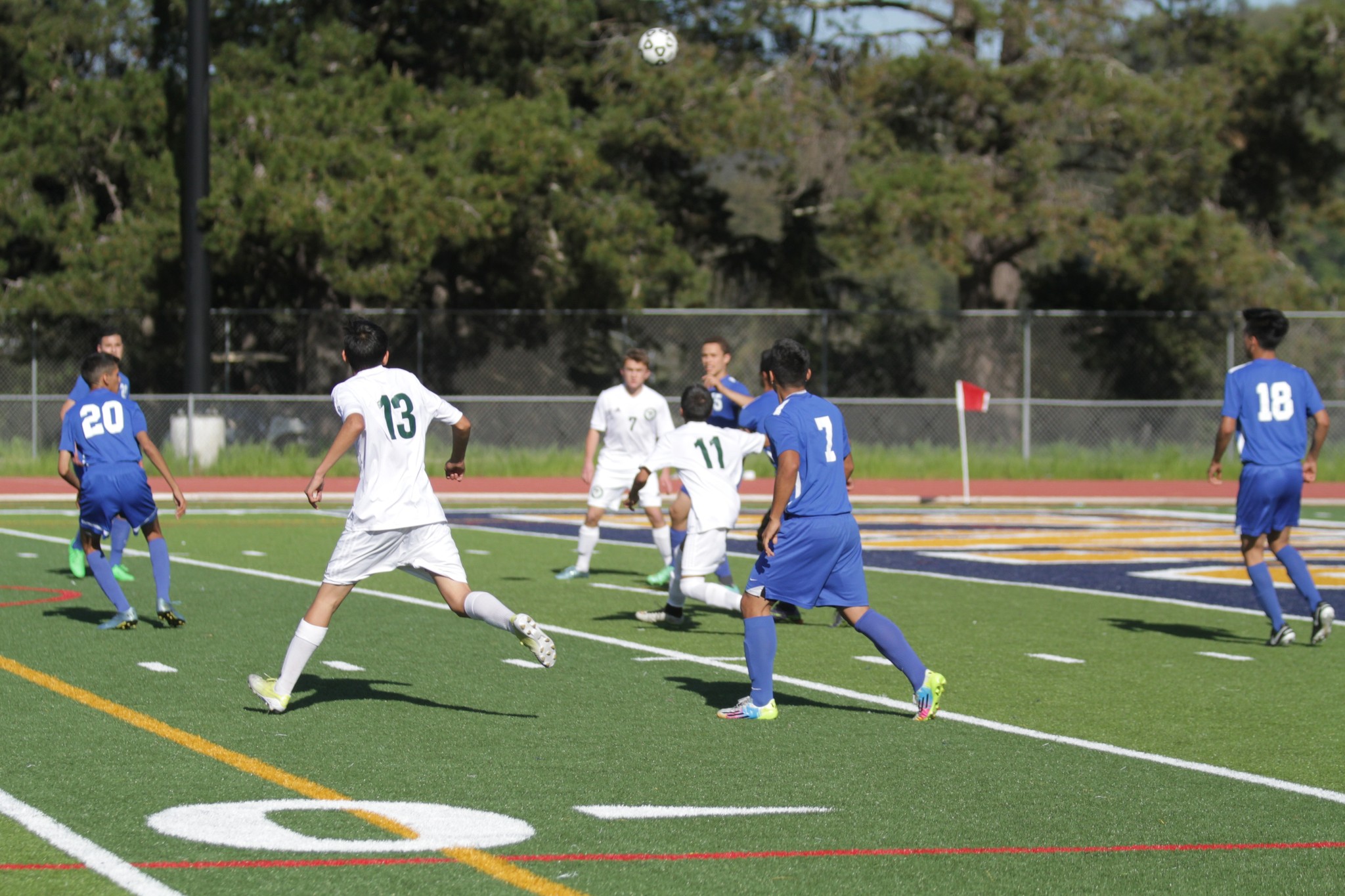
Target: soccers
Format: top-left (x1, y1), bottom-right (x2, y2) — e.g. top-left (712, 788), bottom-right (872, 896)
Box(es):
top-left (637, 27), bottom-right (679, 66)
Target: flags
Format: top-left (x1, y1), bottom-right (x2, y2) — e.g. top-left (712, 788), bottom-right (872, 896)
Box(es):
top-left (954, 378), bottom-right (992, 415)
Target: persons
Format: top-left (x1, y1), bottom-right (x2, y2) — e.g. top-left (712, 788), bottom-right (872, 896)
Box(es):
top-left (551, 336), bottom-right (858, 630)
top-left (58, 327), bottom-right (190, 628)
top-left (711, 337), bottom-right (947, 724)
top-left (241, 321), bottom-right (557, 714)
top-left (1207, 304), bottom-right (1336, 648)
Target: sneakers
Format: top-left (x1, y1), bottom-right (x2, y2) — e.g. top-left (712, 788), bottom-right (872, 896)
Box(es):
top-left (775, 600), bottom-right (802, 624)
top-left (555, 565), bottom-right (590, 580)
top-left (646, 565), bottom-right (675, 585)
top-left (717, 696), bottom-right (778, 720)
top-left (111, 565), bottom-right (133, 582)
top-left (248, 673), bottom-right (290, 712)
top-left (67, 538), bottom-right (86, 578)
top-left (512, 613), bottom-right (556, 668)
top-left (635, 610), bottom-right (685, 625)
top-left (98, 607), bottom-right (139, 630)
top-left (1311, 601), bottom-right (1334, 646)
top-left (913, 669), bottom-right (947, 721)
top-left (1266, 625), bottom-right (1296, 646)
top-left (156, 598), bottom-right (185, 628)
